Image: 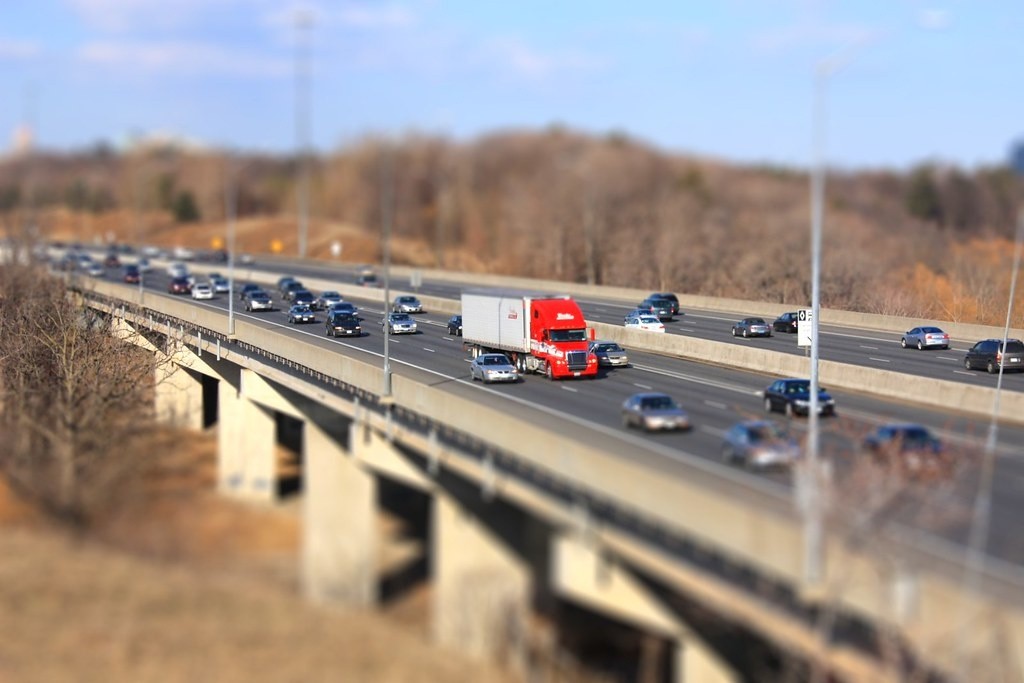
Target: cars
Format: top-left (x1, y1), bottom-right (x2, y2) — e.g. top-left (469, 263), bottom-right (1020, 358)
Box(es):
top-left (773, 313), bottom-right (798, 335)
top-left (901, 326), bottom-right (949, 350)
top-left (621, 393), bottom-right (690, 432)
top-left (624, 292), bottom-right (680, 334)
top-left (44, 239), bottom-right (362, 338)
top-left (392, 295), bottom-right (423, 314)
top-left (719, 420), bottom-right (799, 477)
top-left (447, 315), bottom-right (462, 337)
top-left (863, 424), bottom-right (940, 458)
top-left (732, 318), bottom-right (771, 338)
top-left (382, 313), bottom-right (418, 335)
top-left (470, 353), bottom-right (518, 384)
top-left (588, 341), bottom-right (628, 368)
top-left (764, 378), bottom-right (834, 419)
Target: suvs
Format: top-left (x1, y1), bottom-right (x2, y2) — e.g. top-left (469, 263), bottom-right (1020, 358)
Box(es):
top-left (964, 339), bottom-right (1024, 375)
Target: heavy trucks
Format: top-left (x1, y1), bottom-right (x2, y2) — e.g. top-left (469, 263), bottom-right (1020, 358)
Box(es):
top-left (461, 286), bottom-right (599, 382)
